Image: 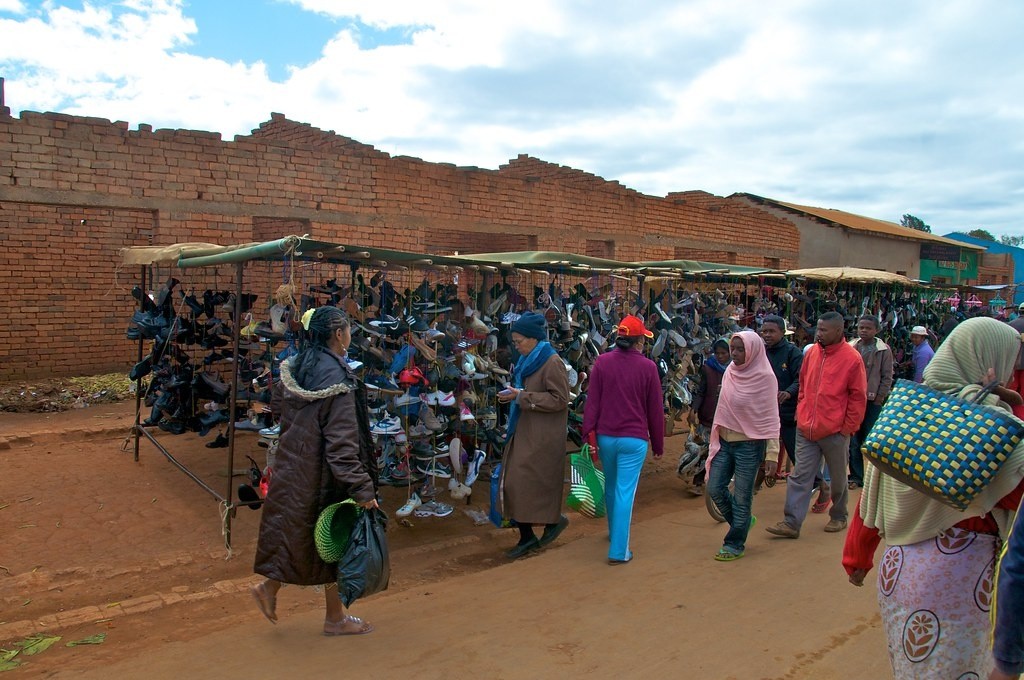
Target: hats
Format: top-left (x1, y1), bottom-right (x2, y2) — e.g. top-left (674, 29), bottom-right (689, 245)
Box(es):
top-left (512, 310), bottom-right (547, 339)
top-left (315, 499), bottom-right (361, 563)
top-left (910, 325), bottom-right (929, 337)
top-left (617, 315), bottom-right (654, 339)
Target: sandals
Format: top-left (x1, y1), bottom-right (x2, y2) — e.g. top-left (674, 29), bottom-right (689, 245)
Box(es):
top-left (748, 513), bottom-right (757, 531)
top-left (321, 615), bottom-right (374, 635)
top-left (249, 581), bottom-right (278, 625)
top-left (714, 548), bottom-right (745, 561)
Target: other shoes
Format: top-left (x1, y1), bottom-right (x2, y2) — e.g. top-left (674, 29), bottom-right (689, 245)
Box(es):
top-left (609, 552), bottom-right (633, 565)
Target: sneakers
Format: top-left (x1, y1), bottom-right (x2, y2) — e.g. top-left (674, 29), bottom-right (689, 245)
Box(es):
top-left (824, 518), bottom-right (848, 531)
top-left (765, 520), bottom-right (800, 539)
top-left (126, 268), bottom-right (1024, 527)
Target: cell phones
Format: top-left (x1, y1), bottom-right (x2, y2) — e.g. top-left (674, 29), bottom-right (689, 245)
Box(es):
top-left (499, 388), bottom-right (512, 396)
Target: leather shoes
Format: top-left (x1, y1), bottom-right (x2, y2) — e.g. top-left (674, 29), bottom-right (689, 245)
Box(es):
top-left (539, 514), bottom-right (569, 546)
top-left (507, 535), bottom-right (539, 557)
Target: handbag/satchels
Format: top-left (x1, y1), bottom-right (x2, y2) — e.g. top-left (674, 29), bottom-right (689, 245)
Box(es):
top-left (565, 443), bottom-right (607, 518)
top-left (859, 377), bottom-right (1024, 512)
top-left (336, 504), bottom-right (390, 609)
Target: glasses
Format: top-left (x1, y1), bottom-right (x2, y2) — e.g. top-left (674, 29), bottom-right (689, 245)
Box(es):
top-left (511, 336), bottom-right (527, 346)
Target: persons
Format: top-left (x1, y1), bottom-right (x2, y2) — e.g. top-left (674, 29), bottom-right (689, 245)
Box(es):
top-left (249, 306), bottom-right (379, 636)
top-left (703, 331), bottom-right (781, 560)
top-left (687, 338), bottom-right (732, 495)
top-left (996, 306), bottom-right (1019, 323)
top-left (766, 311), bottom-right (867, 541)
top-left (909, 326), bottom-right (935, 383)
top-left (841, 316), bottom-right (1024, 680)
top-left (583, 316), bottom-right (664, 566)
top-left (847, 314), bottom-right (893, 489)
top-left (1006, 302), bottom-right (1024, 421)
top-left (762, 316), bottom-right (832, 513)
top-left (437, 299), bottom-right (471, 352)
top-left (939, 314), bottom-right (959, 337)
top-left (497, 312), bottom-right (570, 558)
